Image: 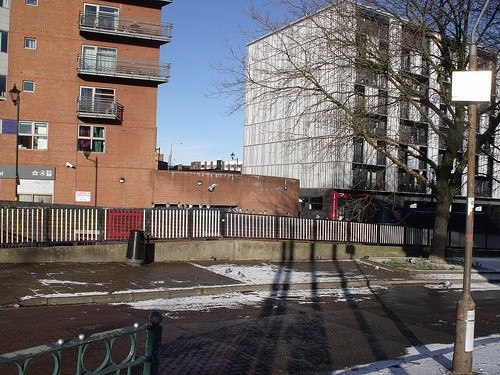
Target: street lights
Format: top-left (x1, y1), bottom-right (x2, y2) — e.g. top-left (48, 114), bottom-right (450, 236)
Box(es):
top-left (169, 142), bottom-right (183, 170)
top-left (82, 145), bottom-right (98, 207)
top-left (8, 82), bottom-right (22, 202)
top-left (230, 152), bottom-right (238, 172)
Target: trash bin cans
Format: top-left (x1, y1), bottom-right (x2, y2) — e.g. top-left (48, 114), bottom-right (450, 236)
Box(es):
top-left (126, 229), bottom-right (146, 264)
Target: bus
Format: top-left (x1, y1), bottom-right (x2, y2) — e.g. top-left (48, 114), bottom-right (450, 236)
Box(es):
top-left (322, 189), bottom-right (500, 233)
